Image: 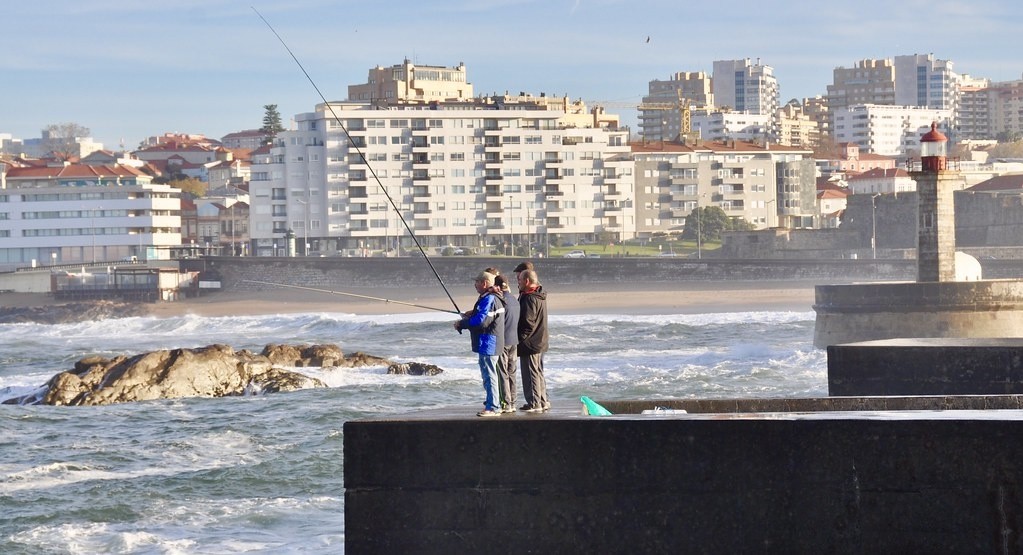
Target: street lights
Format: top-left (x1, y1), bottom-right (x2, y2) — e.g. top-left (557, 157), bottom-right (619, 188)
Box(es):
top-left (698, 194), bottom-right (706, 260)
top-left (872, 192), bottom-right (882, 259)
top-left (623, 198), bottom-right (631, 257)
top-left (509, 196), bottom-right (513, 256)
top-left (93, 206), bottom-right (102, 266)
top-left (296, 198), bottom-right (308, 257)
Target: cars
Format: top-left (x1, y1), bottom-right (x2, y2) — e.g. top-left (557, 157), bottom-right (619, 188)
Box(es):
top-left (562, 250), bottom-right (585, 259)
top-left (588, 254), bottom-right (601, 258)
top-left (412, 252), bottom-right (428, 257)
top-left (658, 250), bottom-right (677, 257)
top-left (442, 247), bottom-right (464, 256)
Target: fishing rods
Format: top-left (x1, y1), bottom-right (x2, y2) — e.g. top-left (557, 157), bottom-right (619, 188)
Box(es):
top-left (247, 4), bottom-right (463, 319)
top-left (243, 279), bottom-right (472, 317)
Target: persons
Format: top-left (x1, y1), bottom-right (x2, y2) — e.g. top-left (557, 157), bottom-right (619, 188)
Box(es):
top-left (513, 260), bottom-right (550, 408)
top-left (485, 267), bottom-right (499, 276)
top-left (516, 269), bottom-right (548, 412)
top-left (494, 275), bottom-right (520, 413)
top-left (453, 271), bottom-right (507, 417)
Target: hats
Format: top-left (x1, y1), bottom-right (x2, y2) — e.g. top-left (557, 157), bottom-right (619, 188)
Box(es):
top-left (470, 271), bottom-right (494, 285)
top-left (495, 275), bottom-right (508, 287)
top-left (513, 262), bottom-right (533, 272)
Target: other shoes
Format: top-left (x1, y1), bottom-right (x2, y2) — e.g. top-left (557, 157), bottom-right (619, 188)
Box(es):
top-left (512, 406), bottom-right (519, 411)
top-left (476, 409), bottom-right (501, 417)
top-left (519, 407), bottom-right (543, 412)
top-left (502, 405), bottom-right (512, 413)
top-left (483, 401), bottom-right (488, 405)
top-left (523, 403), bottom-right (548, 410)
